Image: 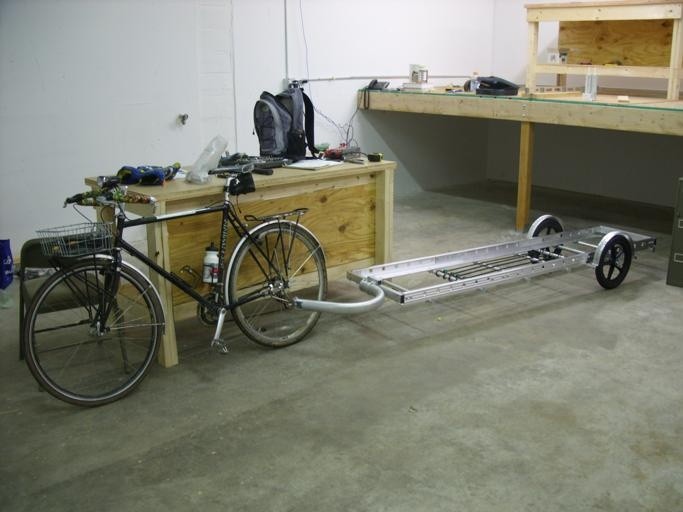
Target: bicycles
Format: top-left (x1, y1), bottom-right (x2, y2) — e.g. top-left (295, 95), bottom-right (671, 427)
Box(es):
top-left (22, 176), bottom-right (327, 408)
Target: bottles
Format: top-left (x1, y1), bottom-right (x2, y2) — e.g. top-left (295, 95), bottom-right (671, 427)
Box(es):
top-left (418, 69), bottom-right (428, 83)
top-left (203, 241), bottom-right (219, 284)
top-left (470, 71), bottom-right (479, 94)
top-left (412, 70), bottom-right (419, 84)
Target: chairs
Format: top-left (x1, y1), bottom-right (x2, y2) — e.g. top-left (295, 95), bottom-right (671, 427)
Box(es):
top-left (19, 231), bottom-right (133, 392)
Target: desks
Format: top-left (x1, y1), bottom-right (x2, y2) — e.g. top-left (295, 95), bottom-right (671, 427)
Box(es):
top-left (523, 0), bottom-right (683, 101)
top-left (357, 85), bottom-right (683, 233)
top-left (84, 154), bottom-right (397, 368)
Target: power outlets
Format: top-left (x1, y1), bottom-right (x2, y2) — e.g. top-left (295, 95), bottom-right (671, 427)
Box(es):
top-left (548, 53), bottom-right (559, 64)
top-left (410, 64), bottom-right (429, 84)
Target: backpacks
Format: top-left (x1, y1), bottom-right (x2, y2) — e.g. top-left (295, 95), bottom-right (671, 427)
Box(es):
top-left (254, 83), bottom-right (320, 162)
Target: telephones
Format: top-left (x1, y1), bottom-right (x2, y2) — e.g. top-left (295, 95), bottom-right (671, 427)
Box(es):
top-left (363, 80), bottom-right (390, 110)
